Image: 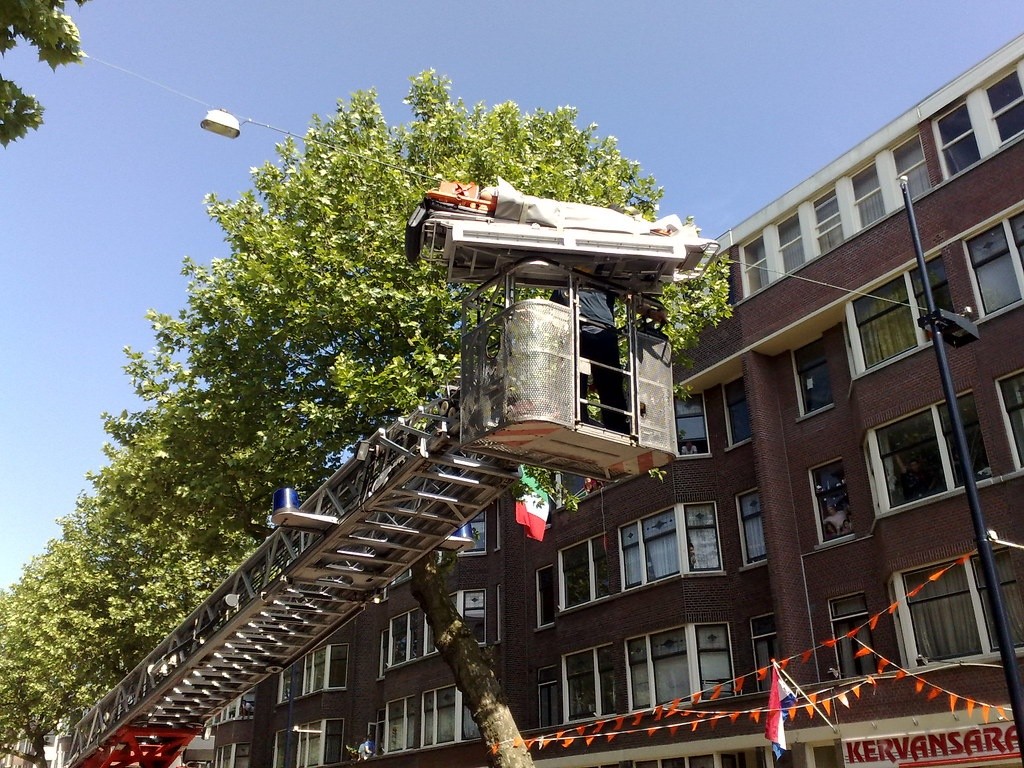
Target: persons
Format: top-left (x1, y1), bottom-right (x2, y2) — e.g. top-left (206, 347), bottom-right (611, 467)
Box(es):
top-left (357, 735), bottom-right (374, 762)
top-left (824, 522), bottom-right (838, 538)
top-left (894, 454), bottom-right (929, 501)
top-left (688, 543), bottom-right (696, 564)
top-left (549, 283), bottom-right (630, 434)
top-left (822, 503), bottom-right (852, 535)
top-left (682, 442), bottom-right (697, 455)
top-left (585, 477), bottom-right (597, 493)
top-left (241, 700), bottom-right (254, 721)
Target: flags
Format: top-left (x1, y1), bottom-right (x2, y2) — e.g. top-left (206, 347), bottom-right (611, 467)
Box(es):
top-left (516, 464), bottom-right (549, 541)
top-left (765, 667), bottom-right (798, 760)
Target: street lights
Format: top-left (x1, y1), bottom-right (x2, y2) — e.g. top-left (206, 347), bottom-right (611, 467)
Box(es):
top-left (900, 172), bottom-right (1023, 768)
